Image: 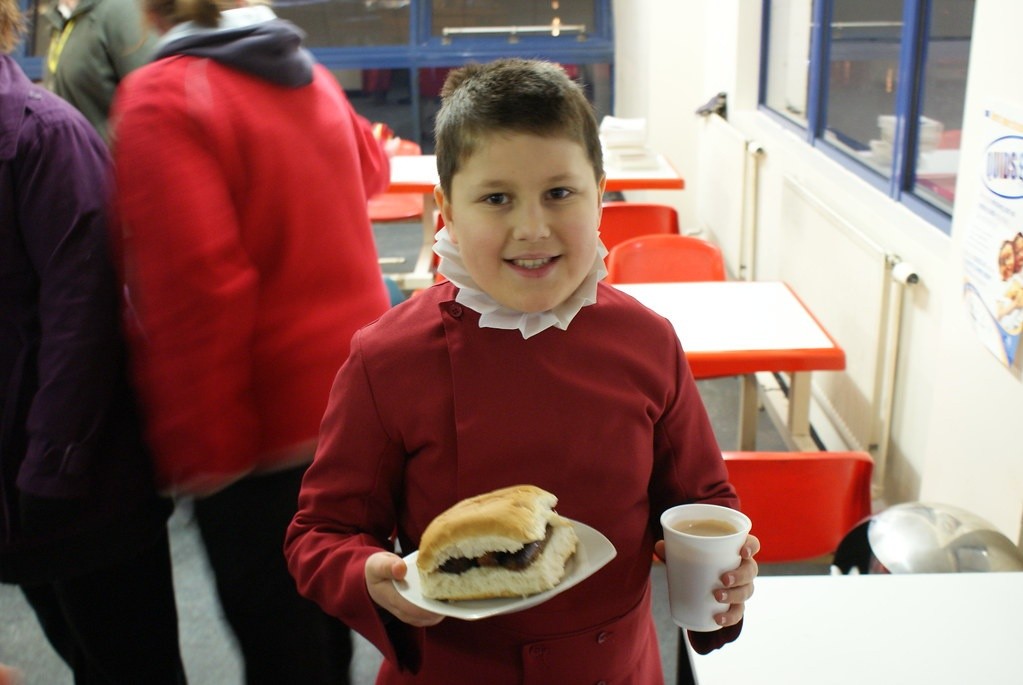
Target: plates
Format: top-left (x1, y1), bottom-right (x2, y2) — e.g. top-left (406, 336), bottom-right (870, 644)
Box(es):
top-left (392, 516), bottom-right (616, 622)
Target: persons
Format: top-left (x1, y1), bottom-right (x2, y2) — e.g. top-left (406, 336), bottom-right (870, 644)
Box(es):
top-left (112, 1), bottom-right (391, 685)
top-left (285, 56), bottom-right (759, 684)
top-left (40, 0), bottom-right (163, 135)
top-left (0, 0), bottom-right (185, 684)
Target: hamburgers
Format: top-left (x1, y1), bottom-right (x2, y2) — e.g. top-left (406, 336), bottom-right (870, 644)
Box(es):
top-left (416, 484), bottom-right (578, 601)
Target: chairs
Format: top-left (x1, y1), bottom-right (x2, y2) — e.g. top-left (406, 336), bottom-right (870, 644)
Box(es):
top-left (653, 450), bottom-right (875, 566)
top-left (367, 139), bottom-right (424, 223)
top-left (600, 201), bottom-right (680, 269)
top-left (608, 234), bottom-right (727, 285)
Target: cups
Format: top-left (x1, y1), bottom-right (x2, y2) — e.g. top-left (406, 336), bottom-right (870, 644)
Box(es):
top-left (660, 504), bottom-right (752, 632)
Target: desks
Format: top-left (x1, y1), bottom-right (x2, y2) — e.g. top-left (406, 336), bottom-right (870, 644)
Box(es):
top-left (388, 155), bottom-right (440, 239)
top-left (676, 571), bottom-right (1023, 685)
top-left (610, 279), bottom-right (848, 449)
top-left (386, 143), bottom-right (685, 191)
